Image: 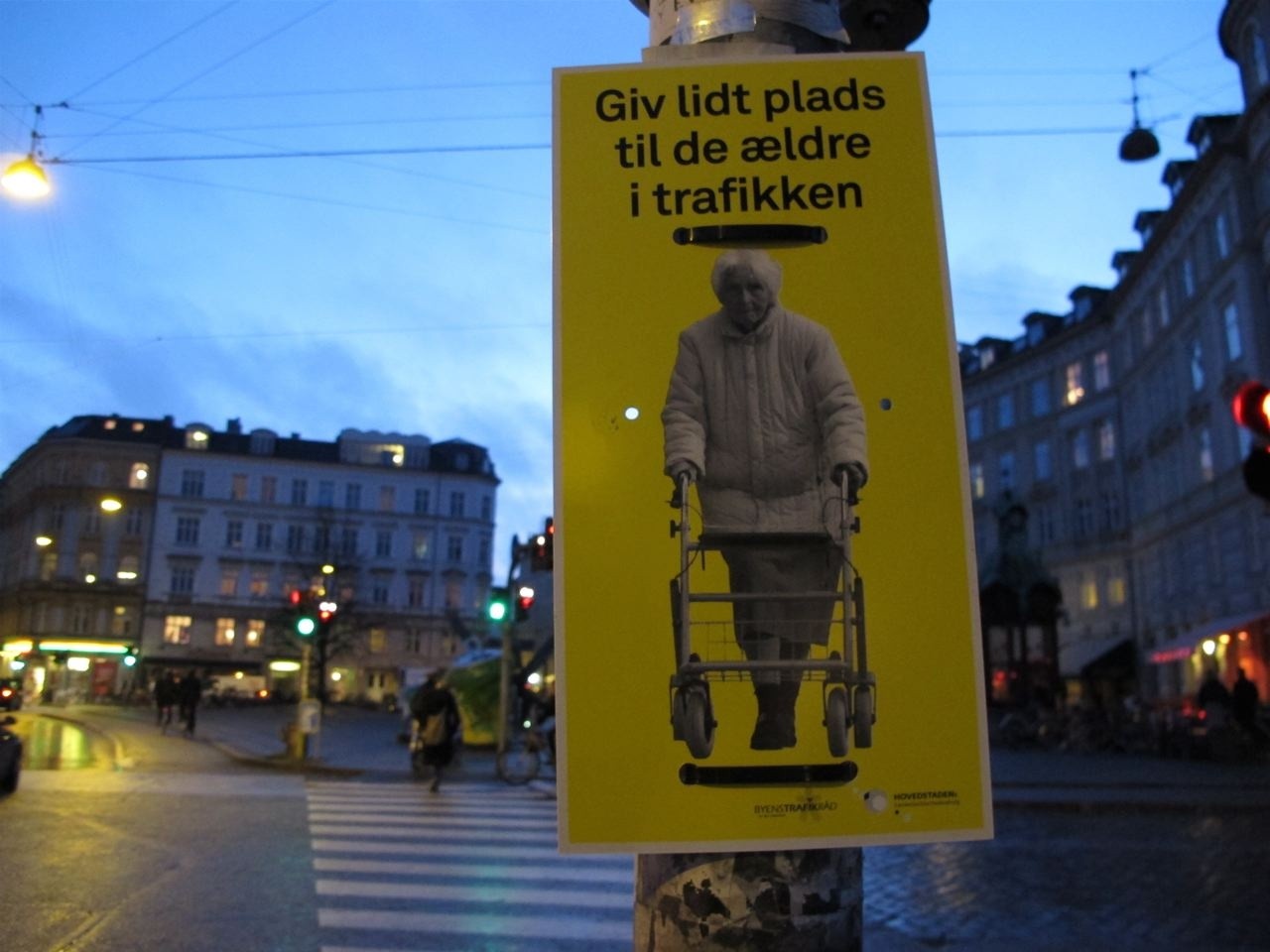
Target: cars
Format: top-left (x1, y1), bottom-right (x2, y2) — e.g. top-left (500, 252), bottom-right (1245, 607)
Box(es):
top-left (0, 676), bottom-right (25, 795)
top-left (207, 660), bottom-right (275, 707)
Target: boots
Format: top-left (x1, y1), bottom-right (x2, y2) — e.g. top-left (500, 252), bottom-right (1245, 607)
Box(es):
top-left (749, 680), bottom-right (801, 750)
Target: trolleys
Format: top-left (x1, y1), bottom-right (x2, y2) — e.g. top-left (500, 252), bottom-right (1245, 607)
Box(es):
top-left (664, 483), bottom-right (876, 757)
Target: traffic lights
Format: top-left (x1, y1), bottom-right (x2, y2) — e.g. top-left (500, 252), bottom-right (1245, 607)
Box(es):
top-left (530, 535), bottom-right (549, 573)
top-left (546, 517), bottom-right (553, 562)
top-left (515, 584), bottom-right (536, 622)
top-left (1234, 379), bottom-right (1270, 499)
top-left (486, 586), bottom-right (509, 625)
top-left (294, 590), bottom-right (318, 638)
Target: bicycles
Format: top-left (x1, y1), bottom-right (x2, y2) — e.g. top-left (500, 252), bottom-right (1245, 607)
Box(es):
top-left (495, 716), bottom-right (557, 787)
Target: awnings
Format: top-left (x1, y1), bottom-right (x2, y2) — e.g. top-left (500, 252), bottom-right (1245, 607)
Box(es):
top-left (1060, 632), bottom-right (1133, 678)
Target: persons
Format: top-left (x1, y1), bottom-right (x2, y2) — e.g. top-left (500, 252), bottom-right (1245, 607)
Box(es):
top-left (153, 667), bottom-right (203, 737)
top-left (661, 249), bottom-right (869, 750)
top-left (1195, 668), bottom-right (1261, 762)
top-left (409, 667), bottom-right (557, 793)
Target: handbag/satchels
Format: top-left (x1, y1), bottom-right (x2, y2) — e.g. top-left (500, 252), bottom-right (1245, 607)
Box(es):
top-left (421, 709), bottom-right (447, 746)
